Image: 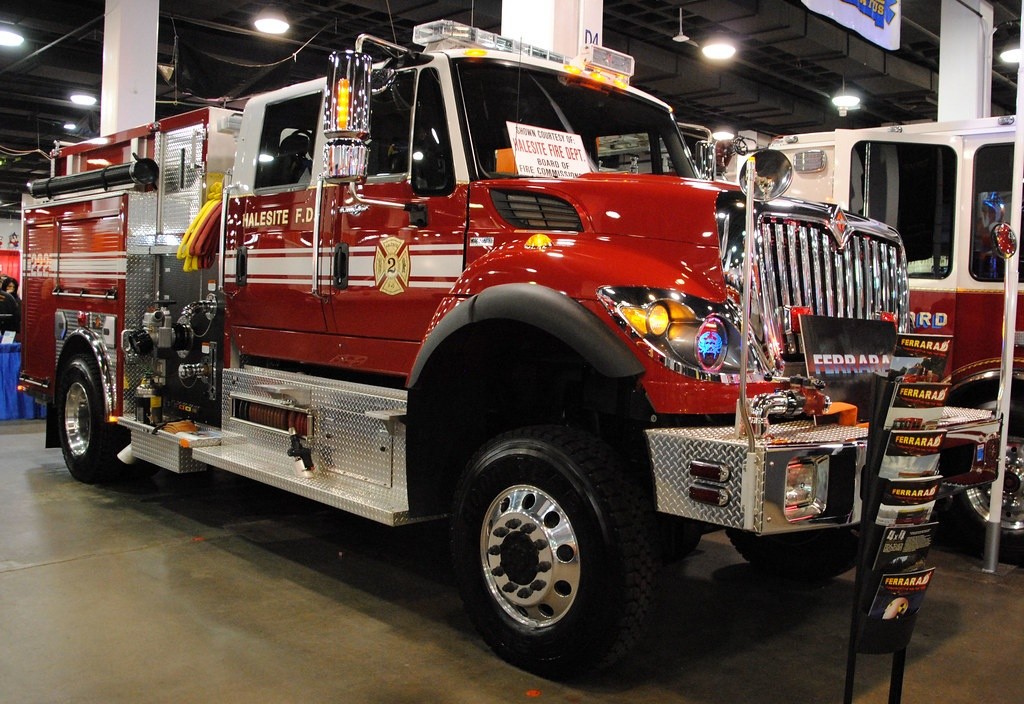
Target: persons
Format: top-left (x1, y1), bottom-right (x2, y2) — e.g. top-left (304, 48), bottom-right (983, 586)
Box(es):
top-left (0, 274), bottom-right (22, 333)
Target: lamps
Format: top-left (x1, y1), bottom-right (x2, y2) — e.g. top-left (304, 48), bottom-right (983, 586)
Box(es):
top-left (672, 8), bottom-right (689, 42)
top-left (832, 72), bottom-right (860, 109)
top-left (701, 28), bottom-right (735, 60)
top-left (253, 4), bottom-right (290, 35)
top-left (712, 123), bottom-right (735, 141)
top-left (999, 36), bottom-right (1020, 63)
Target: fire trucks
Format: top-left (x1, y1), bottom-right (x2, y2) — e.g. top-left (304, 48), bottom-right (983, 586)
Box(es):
top-left (14, 16), bottom-right (1005, 667)
top-left (675, 112), bottom-right (1024, 569)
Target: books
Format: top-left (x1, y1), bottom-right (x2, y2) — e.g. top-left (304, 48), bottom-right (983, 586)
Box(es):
top-left (867, 332), bottom-right (954, 620)
